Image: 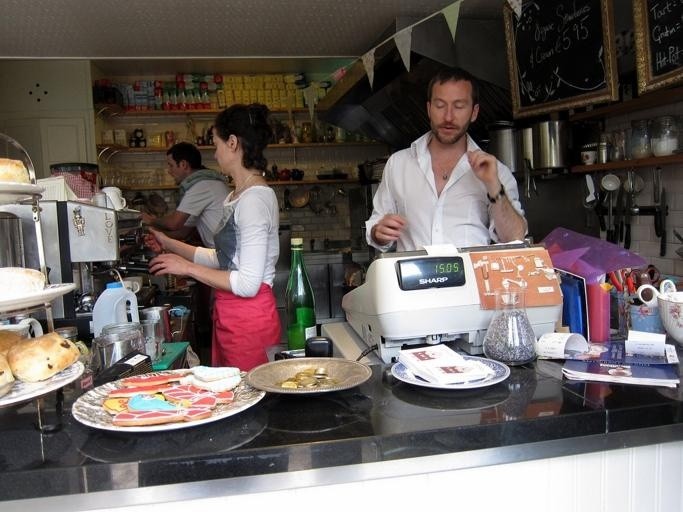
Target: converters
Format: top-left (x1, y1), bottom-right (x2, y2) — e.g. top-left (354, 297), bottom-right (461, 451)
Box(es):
top-left (305, 336), bottom-right (333, 357)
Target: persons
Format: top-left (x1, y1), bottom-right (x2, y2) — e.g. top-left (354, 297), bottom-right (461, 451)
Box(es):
top-left (143, 103), bottom-right (281, 371)
top-left (138, 142), bottom-right (232, 354)
top-left (364, 70), bottom-right (529, 254)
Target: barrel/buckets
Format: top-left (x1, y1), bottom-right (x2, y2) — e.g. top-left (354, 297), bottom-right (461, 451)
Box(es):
top-left (92, 282), bottom-right (141, 338)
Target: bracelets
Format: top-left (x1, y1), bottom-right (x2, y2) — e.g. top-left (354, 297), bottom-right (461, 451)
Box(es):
top-left (486, 185), bottom-right (505, 205)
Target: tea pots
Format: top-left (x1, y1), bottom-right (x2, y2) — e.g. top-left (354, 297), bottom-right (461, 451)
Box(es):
top-left (276, 168), bottom-right (291, 180)
top-left (288, 168), bottom-right (305, 180)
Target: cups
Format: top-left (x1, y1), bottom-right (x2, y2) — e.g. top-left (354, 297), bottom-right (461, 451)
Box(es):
top-left (599, 169), bottom-right (621, 193)
top-left (99, 171), bottom-right (170, 186)
top-left (140, 320), bottom-right (163, 365)
top-left (629, 305), bottom-right (666, 334)
top-left (586, 283), bottom-right (610, 343)
top-left (141, 306), bottom-right (184, 343)
top-left (101, 185), bottom-right (126, 212)
top-left (624, 175), bottom-right (645, 193)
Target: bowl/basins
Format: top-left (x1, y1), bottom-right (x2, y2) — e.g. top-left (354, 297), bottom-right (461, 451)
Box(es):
top-left (580, 151), bottom-right (598, 166)
top-left (657, 291), bottom-right (683, 346)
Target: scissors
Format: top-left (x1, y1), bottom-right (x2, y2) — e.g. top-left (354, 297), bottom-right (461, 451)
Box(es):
top-left (609, 266), bottom-right (660, 304)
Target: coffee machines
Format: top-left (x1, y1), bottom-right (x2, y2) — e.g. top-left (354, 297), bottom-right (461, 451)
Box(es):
top-left (1, 200), bottom-right (120, 337)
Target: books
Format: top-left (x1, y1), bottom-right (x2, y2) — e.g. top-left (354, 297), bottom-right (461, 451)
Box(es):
top-left (561, 341), bottom-right (681, 389)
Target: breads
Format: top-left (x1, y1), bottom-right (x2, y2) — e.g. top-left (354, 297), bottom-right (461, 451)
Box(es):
top-left (0, 329), bottom-right (24, 356)
top-left (8, 332), bottom-right (81, 384)
top-left (0, 354), bottom-right (14, 399)
top-left (0, 158), bottom-right (28, 184)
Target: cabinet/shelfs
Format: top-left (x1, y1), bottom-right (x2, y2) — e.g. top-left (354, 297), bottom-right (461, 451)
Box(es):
top-left (567, 86), bottom-right (683, 228)
top-left (95, 108), bottom-right (389, 190)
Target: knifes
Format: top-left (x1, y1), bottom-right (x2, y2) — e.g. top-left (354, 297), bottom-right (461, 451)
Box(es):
top-left (613, 167), bottom-right (672, 257)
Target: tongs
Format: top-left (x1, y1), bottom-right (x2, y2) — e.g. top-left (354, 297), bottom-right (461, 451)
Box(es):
top-left (523, 158), bottom-right (539, 203)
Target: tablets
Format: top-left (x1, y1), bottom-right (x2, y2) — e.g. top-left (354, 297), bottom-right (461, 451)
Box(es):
top-left (553, 267), bottom-right (588, 342)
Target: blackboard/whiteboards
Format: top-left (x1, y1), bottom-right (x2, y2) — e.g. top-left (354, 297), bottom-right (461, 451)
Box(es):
top-left (632, 0), bottom-right (683, 98)
top-left (504, 0), bottom-right (620, 120)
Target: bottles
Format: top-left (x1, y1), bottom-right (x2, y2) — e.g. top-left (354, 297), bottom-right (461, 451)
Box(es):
top-left (597, 115), bottom-right (679, 164)
top-left (102, 322), bottom-right (146, 370)
top-left (271, 160), bottom-right (278, 181)
top-left (285, 238), bottom-right (317, 351)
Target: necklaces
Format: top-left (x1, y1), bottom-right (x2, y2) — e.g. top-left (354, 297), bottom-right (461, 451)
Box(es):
top-left (436, 150), bottom-right (464, 180)
top-left (231, 171), bottom-right (264, 194)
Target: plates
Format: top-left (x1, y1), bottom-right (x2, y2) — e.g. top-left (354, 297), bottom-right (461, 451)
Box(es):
top-left (393, 356), bottom-right (510, 390)
top-left (57, 327), bottom-right (79, 344)
top-left (74, 369), bottom-right (265, 432)
top-left (288, 187), bottom-right (309, 207)
top-left (0, 183), bottom-right (46, 202)
top-left (0, 266), bottom-right (76, 312)
top-left (0, 360), bottom-right (84, 407)
top-left (249, 358), bottom-right (370, 394)
